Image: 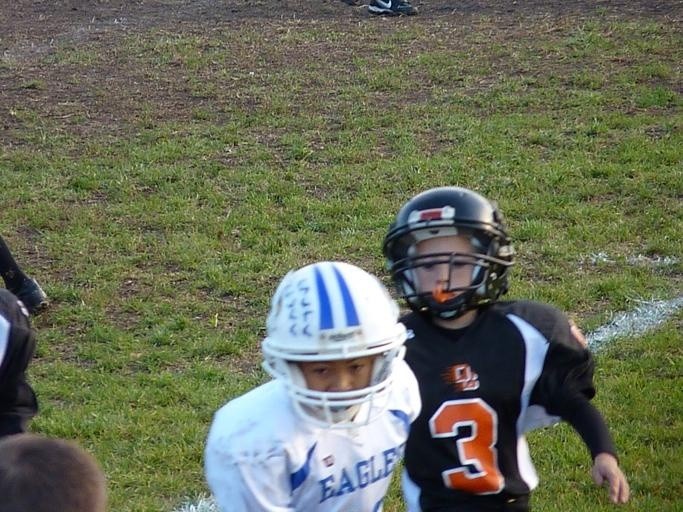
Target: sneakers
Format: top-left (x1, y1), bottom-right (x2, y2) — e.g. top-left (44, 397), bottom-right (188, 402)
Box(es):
top-left (368, 0), bottom-right (418, 16)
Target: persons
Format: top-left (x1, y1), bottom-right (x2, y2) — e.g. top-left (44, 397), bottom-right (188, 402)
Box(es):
top-left (0, 286), bottom-right (39, 436)
top-left (0, 435), bottom-right (107, 512)
top-left (339, 0), bottom-right (419, 16)
top-left (0, 234), bottom-right (49, 314)
top-left (380, 185), bottom-right (631, 512)
top-left (202, 260), bottom-right (422, 512)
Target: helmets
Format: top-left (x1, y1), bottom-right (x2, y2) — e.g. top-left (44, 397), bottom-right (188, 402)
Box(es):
top-left (382, 187), bottom-right (516, 320)
top-left (260, 260), bottom-right (407, 432)
